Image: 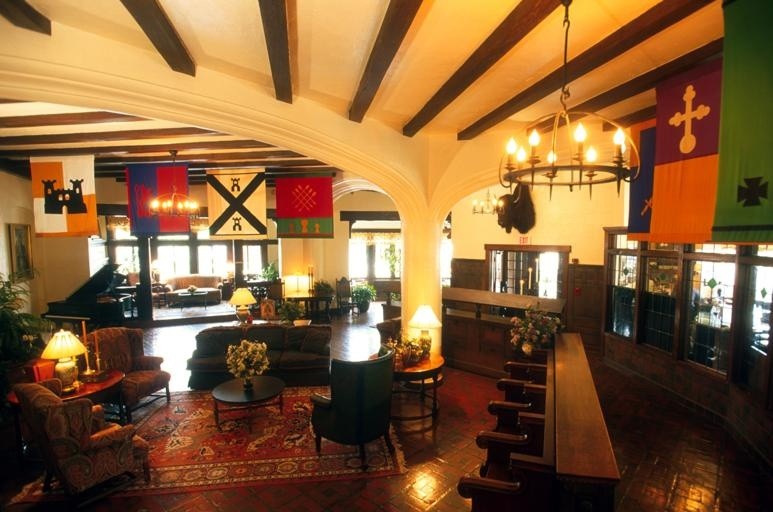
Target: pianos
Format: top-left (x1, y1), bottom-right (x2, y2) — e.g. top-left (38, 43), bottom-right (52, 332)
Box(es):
top-left (39, 267), bottom-right (136, 328)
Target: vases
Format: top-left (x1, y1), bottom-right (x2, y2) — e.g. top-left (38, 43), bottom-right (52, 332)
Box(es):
top-left (243, 372), bottom-right (254, 388)
top-left (522, 340), bottom-right (533, 357)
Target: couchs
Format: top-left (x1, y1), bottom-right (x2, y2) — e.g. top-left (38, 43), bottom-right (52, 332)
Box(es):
top-left (186, 327), bottom-right (332, 389)
top-left (128, 271), bottom-right (223, 311)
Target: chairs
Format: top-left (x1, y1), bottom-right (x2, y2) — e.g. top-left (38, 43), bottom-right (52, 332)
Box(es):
top-left (336, 276), bottom-right (360, 318)
top-left (11, 327), bottom-right (171, 512)
top-left (309, 318), bottom-right (402, 466)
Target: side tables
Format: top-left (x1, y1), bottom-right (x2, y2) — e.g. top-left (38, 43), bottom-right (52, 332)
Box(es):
top-left (369, 348), bottom-right (446, 428)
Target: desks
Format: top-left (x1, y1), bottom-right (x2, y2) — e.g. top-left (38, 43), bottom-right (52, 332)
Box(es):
top-left (228, 320), bottom-right (312, 327)
top-left (287, 296), bottom-right (336, 320)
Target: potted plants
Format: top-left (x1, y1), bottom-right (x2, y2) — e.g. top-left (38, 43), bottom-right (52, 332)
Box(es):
top-left (353, 281), bottom-right (378, 313)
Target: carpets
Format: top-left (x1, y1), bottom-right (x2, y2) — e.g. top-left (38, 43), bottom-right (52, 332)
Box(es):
top-left (7, 385), bottom-right (410, 504)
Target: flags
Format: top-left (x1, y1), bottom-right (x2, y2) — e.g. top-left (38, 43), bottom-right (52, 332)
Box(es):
top-left (205, 169), bottom-right (269, 241)
top-left (625, 118), bottom-right (655, 241)
top-left (272, 171), bottom-right (336, 238)
top-left (648, 56), bottom-right (721, 244)
top-left (710, 0), bottom-right (773, 243)
top-left (29, 154), bottom-right (99, 238)
top-left (125, 163), bottom-right (192, 236)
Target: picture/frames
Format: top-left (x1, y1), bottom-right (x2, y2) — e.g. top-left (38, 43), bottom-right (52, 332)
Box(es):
top-left (9, 223), bottom-right (34, 284)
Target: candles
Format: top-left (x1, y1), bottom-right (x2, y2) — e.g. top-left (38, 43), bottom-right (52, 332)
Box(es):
top-left (519, 257), bottom-right (539, 295)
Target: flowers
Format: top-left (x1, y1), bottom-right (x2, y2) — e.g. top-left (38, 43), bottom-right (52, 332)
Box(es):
top-left (510, 305), bottom-right (561, 346)
top-left (226, 340), bottom-right (271, 379)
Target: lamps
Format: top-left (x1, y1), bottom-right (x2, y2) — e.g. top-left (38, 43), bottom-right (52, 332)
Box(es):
top-left (228, 288), bottom-right (257, 326)
top-left (473, 0), bottom-right (640, 213)
top-left (409, 304), bottom-right (443, 359)
top-left (149, 150), bottom-right (200, 218)
